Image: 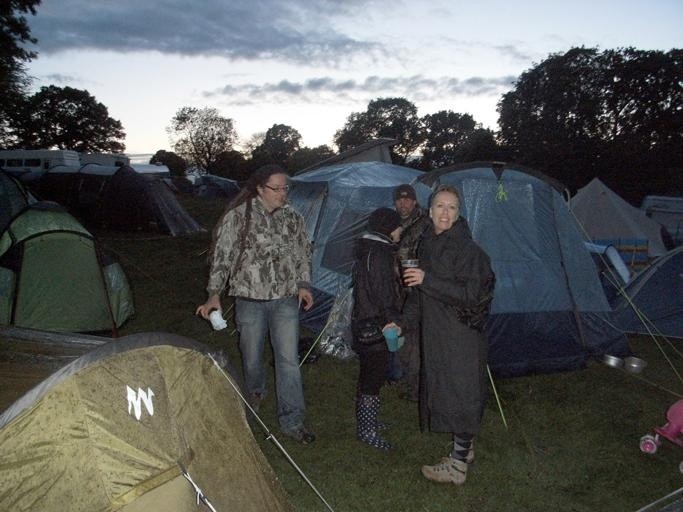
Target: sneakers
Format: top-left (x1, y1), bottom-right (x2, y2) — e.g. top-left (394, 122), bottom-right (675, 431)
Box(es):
top-left (289, 424), bottom-right (315, 445)
top-left (246, 393), bottom-right (261, 418)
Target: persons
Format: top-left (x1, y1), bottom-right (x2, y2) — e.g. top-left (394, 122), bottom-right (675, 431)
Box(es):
top-left (196, 162), bottom-right (315, 446)
top-left (351, 206), bottom-right (411, 450)
top-left (380, 184), bottom-right (496, 485)
top-left (390, 184), bottom-right (433, 405)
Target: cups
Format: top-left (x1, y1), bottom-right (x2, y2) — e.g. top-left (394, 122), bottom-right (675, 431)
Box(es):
top-left (384, 325), bottom-right (403, 354)
top-left (400, 258), bottom-right (422, 289)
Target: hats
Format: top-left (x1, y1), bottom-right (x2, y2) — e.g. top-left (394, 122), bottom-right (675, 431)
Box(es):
top-left (367, 208), bottom-right (402, 233)
top-left (392, 184), bottom-right (417, 201)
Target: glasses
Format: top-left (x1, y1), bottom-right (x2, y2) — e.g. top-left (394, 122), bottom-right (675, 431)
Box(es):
top-left (263, 184), bottom-right (291, 192)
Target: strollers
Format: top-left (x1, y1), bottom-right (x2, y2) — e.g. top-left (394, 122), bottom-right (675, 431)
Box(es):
top-left (638, 398), bottom-right (683, 473)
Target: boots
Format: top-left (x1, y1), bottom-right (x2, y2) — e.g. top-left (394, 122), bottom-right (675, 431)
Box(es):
top-left (357, 392), bottom-right (391, 451)
top-left (435, 449), bottom-right (474, 467)
top-left (421, 458), bottom-right (467, 486)
top-left (356, 378), bottom-right (392, 431)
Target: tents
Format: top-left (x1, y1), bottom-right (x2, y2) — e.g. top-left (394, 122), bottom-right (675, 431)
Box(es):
top-left (282, 159), bottom-right (682, 379)
top-left (0, 332), bottom-right (298, 512)
top-left (0, 168), bottom-right (241, 345)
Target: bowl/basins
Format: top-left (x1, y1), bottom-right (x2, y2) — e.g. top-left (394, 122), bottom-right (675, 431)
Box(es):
top-left (603, 353), bottom-right (624, 369)
top-left (625, 356), bottom-right (648, 374)
